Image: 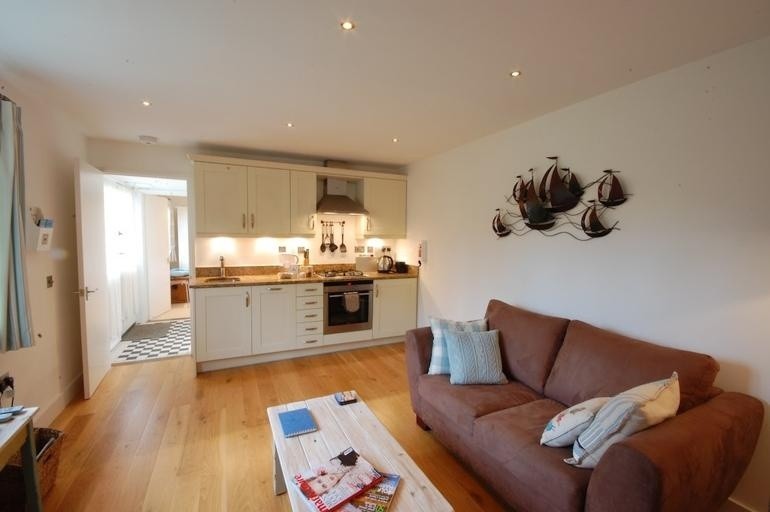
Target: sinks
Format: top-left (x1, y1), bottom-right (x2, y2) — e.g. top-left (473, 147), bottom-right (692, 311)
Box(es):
top-left (204, 277), bottom-right (240, 284)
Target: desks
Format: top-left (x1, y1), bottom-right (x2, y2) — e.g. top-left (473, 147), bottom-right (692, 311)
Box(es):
top-left (0, 404), bottom-right (46, 512)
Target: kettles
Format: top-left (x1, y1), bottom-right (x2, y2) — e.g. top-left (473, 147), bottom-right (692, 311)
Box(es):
top-left (377, 256), bottom-right (393, 273)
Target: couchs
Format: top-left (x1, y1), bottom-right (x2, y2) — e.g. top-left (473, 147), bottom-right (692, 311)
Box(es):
top-left (403, 295), bottom-right (766, 511)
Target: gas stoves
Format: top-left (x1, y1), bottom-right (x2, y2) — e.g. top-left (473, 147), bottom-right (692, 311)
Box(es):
top-left (314, 269), bottom-right (365, 281)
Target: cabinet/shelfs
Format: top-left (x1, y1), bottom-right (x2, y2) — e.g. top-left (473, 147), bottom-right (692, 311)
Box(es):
top-left (203, 163), bottom-right (291, 238)
top-left (290, 169), bottom-right (317, 236)
top-left (364, 178), bottom-right (407, 236)
top-left (373, 279), bottom-right (419, 340)
top-left (253, 285), bottom-right (296, 356)
top-left (295, 284), bottom-right (323, 350)
top-left (195, 287), bottom-right (252, 363)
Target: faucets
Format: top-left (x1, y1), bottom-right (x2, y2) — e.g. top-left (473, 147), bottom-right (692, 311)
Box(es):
top-left (219, 256), bottom-right (225, 277)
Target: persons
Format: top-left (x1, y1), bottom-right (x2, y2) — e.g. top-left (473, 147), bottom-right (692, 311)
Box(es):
top-left (299, 451), bottom-right (366, 500)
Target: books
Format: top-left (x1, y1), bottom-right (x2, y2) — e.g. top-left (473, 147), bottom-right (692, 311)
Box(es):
top-left (335, 391), bottom-right (357, 405)
top-left (334, 472), bottom-right (403, 512)
top-left (289, 444), bottom-right (383, 512)
top-left (276, 407), bottom-right (319, 439)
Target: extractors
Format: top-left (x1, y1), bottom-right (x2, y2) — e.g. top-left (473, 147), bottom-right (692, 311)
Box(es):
top-left (315, 176), bottom-right (369, 217)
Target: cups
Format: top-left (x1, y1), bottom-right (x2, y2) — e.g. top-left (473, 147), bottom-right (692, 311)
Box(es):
top-left (299, 272), bottom-right (306, 278)
top-left (395, 262), bottom-right (405, 273)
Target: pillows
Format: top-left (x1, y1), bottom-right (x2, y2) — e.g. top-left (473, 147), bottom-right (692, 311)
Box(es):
top-left (424, 313), bottom-right (509, 385)
top-left (538, 369), bottom-right (684, 470)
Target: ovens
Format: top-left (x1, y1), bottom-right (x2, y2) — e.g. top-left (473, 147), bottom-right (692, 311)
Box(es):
top-left (324, 293), bottom-right (373, 333)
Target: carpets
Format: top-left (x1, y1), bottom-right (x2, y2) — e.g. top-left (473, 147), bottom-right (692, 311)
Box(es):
top-left (121, 322), bottom-right (172, 342)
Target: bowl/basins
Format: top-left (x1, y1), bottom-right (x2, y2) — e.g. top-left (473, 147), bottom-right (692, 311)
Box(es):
top-left (278, 272), bottom-right (292, 279)
top-left (300, 266), bottom-right (311, 273)
